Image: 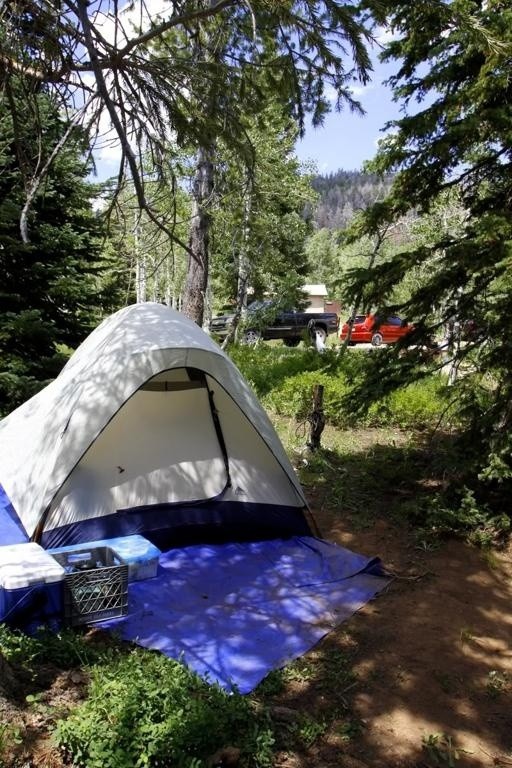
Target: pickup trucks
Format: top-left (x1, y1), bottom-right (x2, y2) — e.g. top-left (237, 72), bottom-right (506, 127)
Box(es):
top-left (206, 298), bottom-right (344, 351)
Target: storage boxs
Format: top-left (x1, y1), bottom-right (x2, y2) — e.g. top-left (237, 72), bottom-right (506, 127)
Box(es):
top-left (0, 542), bottom-right (67, 640)
top-left (44, 534), bottom-right (162, 583)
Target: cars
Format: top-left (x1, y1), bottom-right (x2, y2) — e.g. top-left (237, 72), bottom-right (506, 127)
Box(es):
top-left (338, 309), bottom-right (416, 347)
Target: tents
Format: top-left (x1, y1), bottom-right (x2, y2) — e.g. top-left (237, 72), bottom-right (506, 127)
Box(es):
top-left (0, 300), bottom-right (394, 695)
